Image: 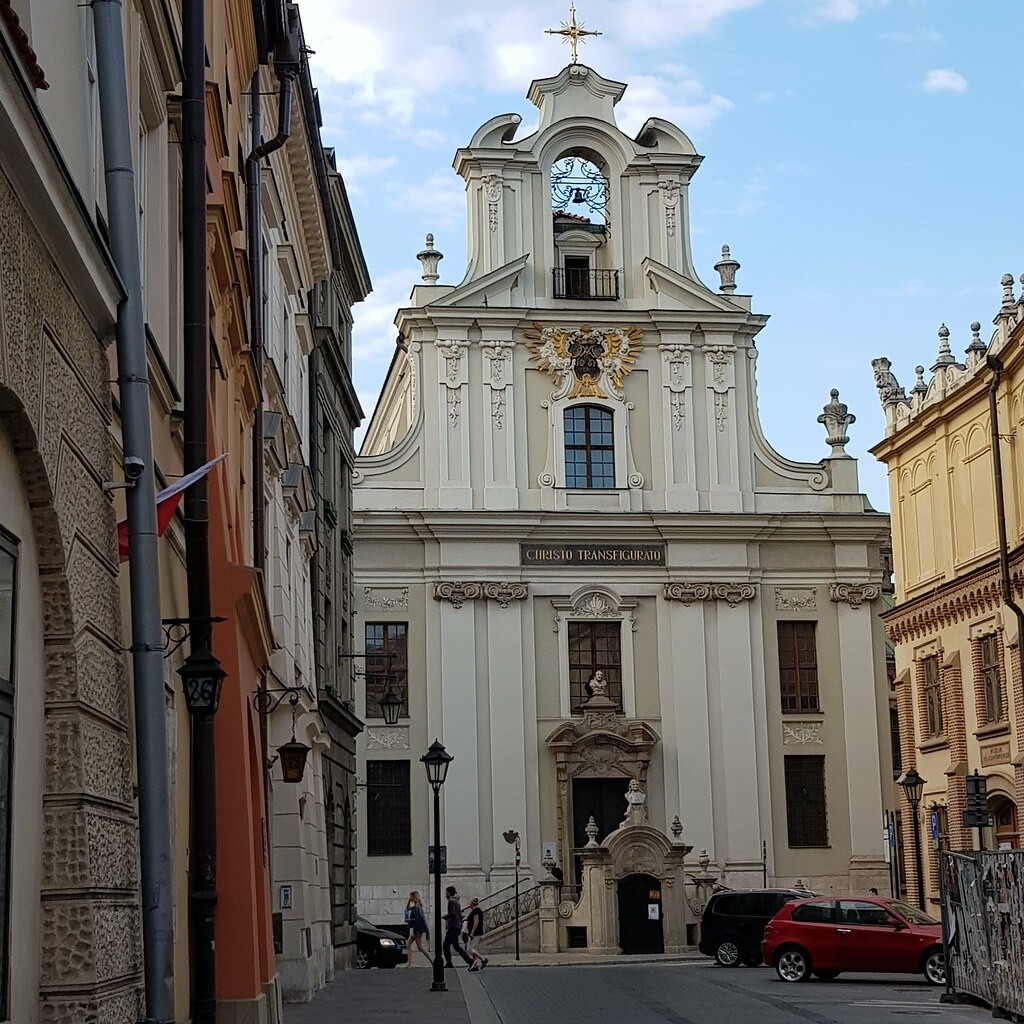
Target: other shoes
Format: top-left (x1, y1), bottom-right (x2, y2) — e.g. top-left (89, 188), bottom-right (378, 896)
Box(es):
top-left (467, 959), bottom-right (477, 971)
top-left (472, 964), bottom-right (479, 972)
top-left (444, 962), bottom-right (454, 968)
top-left (481, 958), bottom-right (489, 969)
top-left (402, 966), bottom-right (412, 969)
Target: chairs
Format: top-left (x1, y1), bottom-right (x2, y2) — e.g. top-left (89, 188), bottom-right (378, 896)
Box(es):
top-left (846, 907), bottom-right (874, 923)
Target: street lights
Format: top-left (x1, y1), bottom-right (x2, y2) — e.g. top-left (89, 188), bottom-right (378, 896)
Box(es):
top-left (419, 738), bottom-right (456, 991)
top-left (895, 766), bottom-right (928, 913)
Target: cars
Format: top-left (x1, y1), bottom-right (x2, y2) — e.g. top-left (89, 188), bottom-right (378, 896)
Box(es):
top-left (358, 909), bottom-right (408, 970)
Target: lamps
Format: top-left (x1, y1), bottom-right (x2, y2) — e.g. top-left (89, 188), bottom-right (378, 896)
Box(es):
top-left (299, 510), bottom-right (315, 532)
top-left (264, 410), bottom-right (283, 439)
top-left (282, 463), bottom-right (303, 486)
top-left (253, 685), bottom-right (312, 783)
top-left (353, 664), bottom-right (403, 725)
top-left (161, 617), bottom-right (231, 719)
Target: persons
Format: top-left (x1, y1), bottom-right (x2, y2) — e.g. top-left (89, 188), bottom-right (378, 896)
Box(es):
top-left (589, 671), bottom-right (607, 695)
top-left (624, 778), bottom-right (647, 804)
top-left (405, 891), bottom-right (434, 967)
top-left (442, 886), bottom-right (489, 971)
top-left (869, 888), bottom-right (879, 896)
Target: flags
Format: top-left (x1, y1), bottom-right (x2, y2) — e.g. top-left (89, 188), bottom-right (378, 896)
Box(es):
top-left (117, 454), bottom-right (225, 563)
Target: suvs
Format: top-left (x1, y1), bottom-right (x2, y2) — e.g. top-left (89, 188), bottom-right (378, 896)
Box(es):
top-left (761, 897), bottom-right (946, 985)
top-left (698, 885), bottom-right (827, 967)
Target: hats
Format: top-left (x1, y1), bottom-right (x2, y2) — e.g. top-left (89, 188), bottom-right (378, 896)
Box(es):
top-left (446, 886), bottom-right (456, 896)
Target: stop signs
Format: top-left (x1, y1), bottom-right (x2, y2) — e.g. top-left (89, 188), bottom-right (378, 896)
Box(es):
top-left (515, 851), bottom-right (521, 864)
